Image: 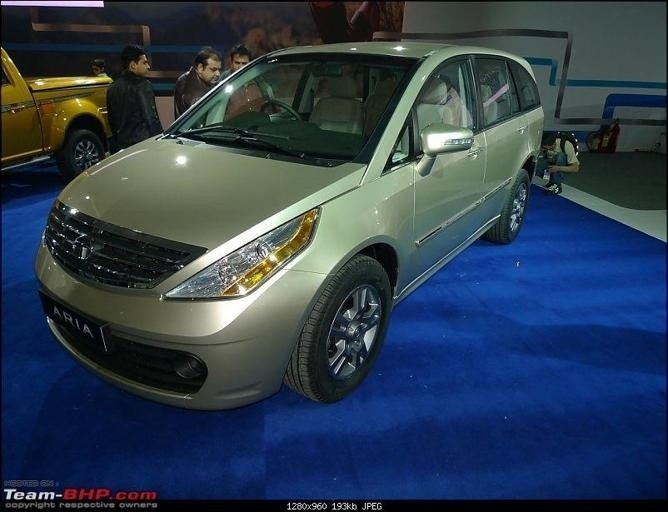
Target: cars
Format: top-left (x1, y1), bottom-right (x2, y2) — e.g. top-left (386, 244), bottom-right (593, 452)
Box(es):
top-left (33, 40), bottom-right (545, 412)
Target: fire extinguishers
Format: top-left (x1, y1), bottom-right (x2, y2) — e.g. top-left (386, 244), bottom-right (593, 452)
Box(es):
top-left (606, 119), bottom-right (620, 154)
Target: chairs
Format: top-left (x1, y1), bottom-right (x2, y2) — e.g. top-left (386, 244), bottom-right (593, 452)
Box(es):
top-left (416, 81), bottom-right (453, 134)
top-left (481, 85), bottom-right (498, 124)
top-left (309, 77), bottom-right (396, 135)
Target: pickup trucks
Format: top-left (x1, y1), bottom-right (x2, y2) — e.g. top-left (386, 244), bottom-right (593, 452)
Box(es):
top-left (0, 46), bottom-right (113, 180)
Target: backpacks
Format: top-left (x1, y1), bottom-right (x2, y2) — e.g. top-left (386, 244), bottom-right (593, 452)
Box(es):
top-left (553, 132), bottom-right (578, 158)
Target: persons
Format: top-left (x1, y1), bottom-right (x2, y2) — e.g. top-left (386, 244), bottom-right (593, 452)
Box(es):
top-left (536, 131), bottom-right (579, 192)
top-left (108, 42), bottom-right (164, 152)
top-left (204, 42), bottom-right (284, 124)
top-left (90, 57), bottom-right (109, 78)
top-left (173, 47), bottom-right (223, 131)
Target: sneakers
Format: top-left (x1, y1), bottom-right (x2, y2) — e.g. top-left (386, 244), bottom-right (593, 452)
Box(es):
top-left (543, 183), bottom-right (562, 196)
